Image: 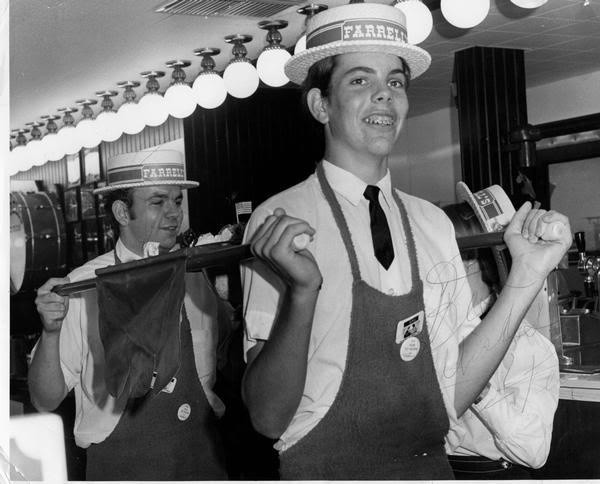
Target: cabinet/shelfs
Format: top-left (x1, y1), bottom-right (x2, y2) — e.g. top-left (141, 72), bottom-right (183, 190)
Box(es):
top-left (56, 181), bottom-right (115, 272)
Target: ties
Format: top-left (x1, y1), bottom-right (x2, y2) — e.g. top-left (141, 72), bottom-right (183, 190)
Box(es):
top-left (363, 184), bottom-right (396, 271)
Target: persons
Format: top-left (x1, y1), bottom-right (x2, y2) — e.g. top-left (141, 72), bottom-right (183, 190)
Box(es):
top-left (238, 3), bottom-right (574, 479)
top-left (26, 150), bottom-right (239, 481)
top-left (429, 179), bottom-right (560, 479)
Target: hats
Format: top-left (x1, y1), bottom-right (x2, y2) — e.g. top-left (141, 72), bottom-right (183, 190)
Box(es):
top-left (89, 145), bottom-right (202, 203)
top-left (452, 175), bottom-right (525, 290)
top-left (281, 0), bottom-right (434, 87)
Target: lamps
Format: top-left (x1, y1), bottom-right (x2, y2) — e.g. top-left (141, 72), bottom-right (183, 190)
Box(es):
top-left (509, 0), bottom-right (549, 10)
top-left (440, 0), bottom-right (490, 29)
top-left (115, 82), bottom-right (146, 135)
top-left (10, 99), bottom-right (102, 177)
top-left (256, 19), bottom-right (294, 88)
top-left (191, 47), bottom-right (228, 110)
top-left (393, 0), bottom-right (433, 46)
top-left (223, 33), bottom-right (260, 99)
top-left (293, 3), bottom-right (328, 57)
top-left (137, 71), bottom-right (168, 127)
top-left (163, 61), bottom-right (198, 119)
top-left (94, 92), bottom-right (123, 143)
